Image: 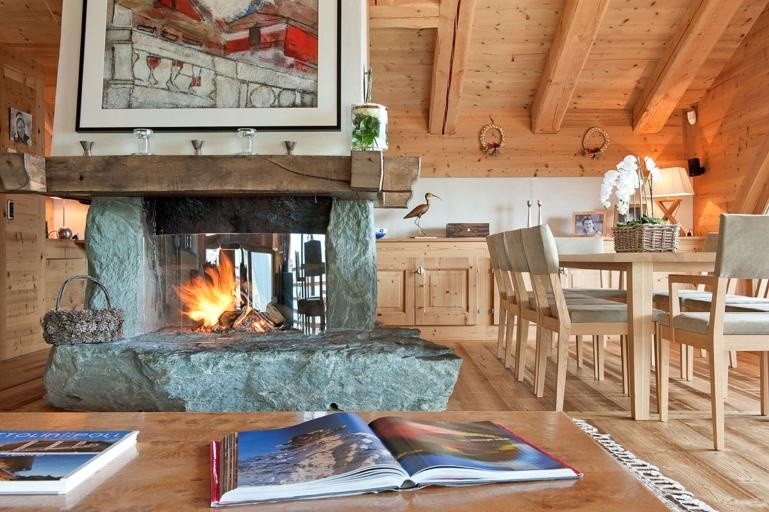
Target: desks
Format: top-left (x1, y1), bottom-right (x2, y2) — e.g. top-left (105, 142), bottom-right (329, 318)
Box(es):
top-left (557, 251), bottom-right (716, 422)
top-left (1, 405), bottom-right (675, 512)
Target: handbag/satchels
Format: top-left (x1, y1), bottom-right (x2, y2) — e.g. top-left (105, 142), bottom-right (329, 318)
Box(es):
top-left (42, 275), bottom-right (123, 344)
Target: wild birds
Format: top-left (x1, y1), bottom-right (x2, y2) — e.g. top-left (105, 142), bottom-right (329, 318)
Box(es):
top-left (403, 192), bottom-right (442, 237)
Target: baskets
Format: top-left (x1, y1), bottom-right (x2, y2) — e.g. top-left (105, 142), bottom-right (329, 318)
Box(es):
top-left (612, 224), bottom-right (680, 253)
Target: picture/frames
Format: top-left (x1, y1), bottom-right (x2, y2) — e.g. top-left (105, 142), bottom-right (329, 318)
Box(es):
top-left (613, 204), bottom-right (646, 228)
top-left (73, 1), bottom-right (345, 132)
top-left (572, 210), bottom-right (607, 237)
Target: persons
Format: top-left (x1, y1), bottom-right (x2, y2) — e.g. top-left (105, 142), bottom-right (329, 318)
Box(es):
top-left (13, 112), bottom-right (31, 147)
top-left (576, 215), bottom-right (602, 235)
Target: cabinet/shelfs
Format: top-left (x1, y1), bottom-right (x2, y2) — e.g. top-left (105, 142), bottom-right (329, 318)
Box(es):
top-left (372, 240), bottom-right (475, 328)
top-left (42, 237), bottom-right (89, 314)
top-left (1, 68), bottom-right (46, 358)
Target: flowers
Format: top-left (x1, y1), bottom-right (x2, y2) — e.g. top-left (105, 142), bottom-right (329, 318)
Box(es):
top-left (478, 124), bottom-right (507, 156)
top-left (582, 126), bottom-right (610, 159)
top-left (600, 154), bottom-right (661, 216)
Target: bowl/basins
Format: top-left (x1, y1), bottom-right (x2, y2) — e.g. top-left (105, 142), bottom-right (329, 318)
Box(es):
top-left (376, 227), bottom-right (389, 241)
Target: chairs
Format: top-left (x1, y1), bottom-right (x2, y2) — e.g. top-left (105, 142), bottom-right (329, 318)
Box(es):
top-left (483, 228), bottom-right (628, 395)
top-left (657, 214), bottom-right (768, 453)
top-left (521, 222), bottom-right (665, 413)
top-left (650, 232), bottom-right (769, 383)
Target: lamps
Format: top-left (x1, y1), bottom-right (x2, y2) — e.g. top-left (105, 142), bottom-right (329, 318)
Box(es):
top-left (643, 168), bottom-right (698, 235)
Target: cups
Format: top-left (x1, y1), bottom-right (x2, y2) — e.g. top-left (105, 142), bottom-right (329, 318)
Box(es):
top-left (80, 141), bottom-right (94, 157)
top-left (237, 129), bottom-right (256, 157)
top-left (133, 128), bottom-right (153, 154)
top-left (191, 140), bottom-right (205, 155)
top-left (284, 141), bottom-right (297, 156)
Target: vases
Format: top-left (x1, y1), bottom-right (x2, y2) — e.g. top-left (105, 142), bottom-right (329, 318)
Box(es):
top-left (350, 103), bottom-right (388, 151)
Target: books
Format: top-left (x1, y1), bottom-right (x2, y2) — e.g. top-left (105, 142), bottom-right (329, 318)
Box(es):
top-left (0, 426), bottom-right (140, 497)
top-left (205, 410), bottom-right (581, 505)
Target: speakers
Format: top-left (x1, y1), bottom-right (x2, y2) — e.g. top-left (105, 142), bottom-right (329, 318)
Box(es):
top-left (688, 158), bottom-right (704, 176)
